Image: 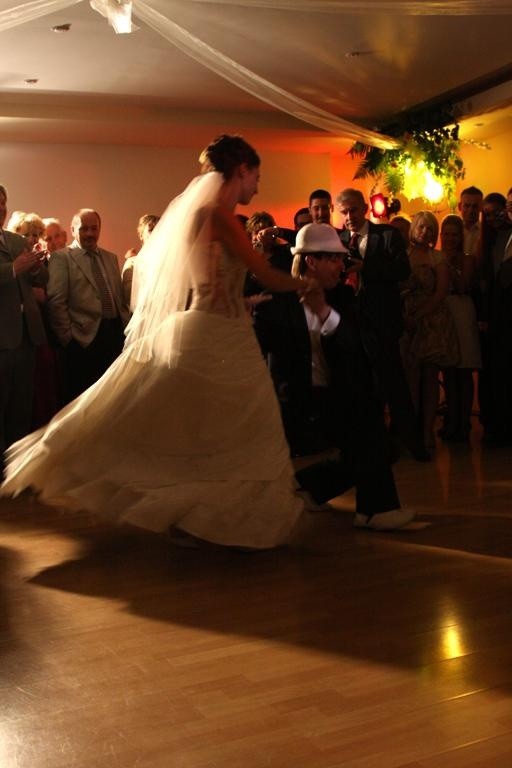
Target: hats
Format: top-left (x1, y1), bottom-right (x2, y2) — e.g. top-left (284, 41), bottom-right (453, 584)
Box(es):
top-left (289, 222), bottom-right (350, 255)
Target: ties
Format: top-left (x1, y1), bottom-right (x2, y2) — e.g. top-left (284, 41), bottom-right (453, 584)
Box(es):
top-left (348, 233), bottom-right (359, 256)
top-left (88, 251), bottom-right (113, 320)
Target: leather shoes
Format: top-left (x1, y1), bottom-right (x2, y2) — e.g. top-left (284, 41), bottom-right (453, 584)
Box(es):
top-left (288, 478), bottom-right (333, 512)
top-left (166, 527), bottom-right (201, 549)
top-left (394, 401), bottom-right (512, 463)
top-left (351, 507), bottom-right (418, 529)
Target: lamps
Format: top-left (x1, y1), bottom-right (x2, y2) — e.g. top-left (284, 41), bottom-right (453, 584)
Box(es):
top-left (368, 192), bottom-right (403, 223)
